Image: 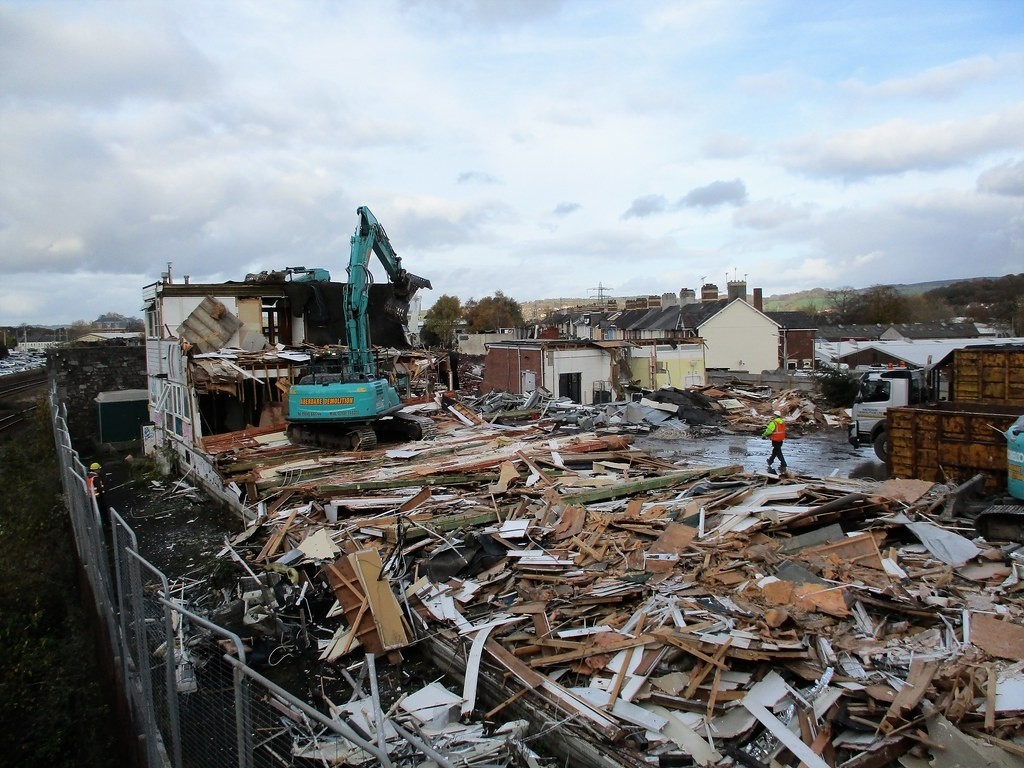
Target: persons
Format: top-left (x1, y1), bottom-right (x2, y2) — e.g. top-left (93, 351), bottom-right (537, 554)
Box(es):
top-left (84, 463), bottom-right (113, 530)
top-left (762, 410), bottom-right (788, 468)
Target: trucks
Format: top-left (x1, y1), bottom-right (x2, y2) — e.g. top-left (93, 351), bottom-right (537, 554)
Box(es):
top-left (848, 347), bottom-right (1022, 463)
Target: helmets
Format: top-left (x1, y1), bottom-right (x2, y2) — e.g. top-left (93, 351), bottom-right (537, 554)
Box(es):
top-left (773, 410), bottom-right (781, 417)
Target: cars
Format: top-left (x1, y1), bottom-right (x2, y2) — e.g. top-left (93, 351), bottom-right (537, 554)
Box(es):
top-left (0, 351), bottom-right (48, 376)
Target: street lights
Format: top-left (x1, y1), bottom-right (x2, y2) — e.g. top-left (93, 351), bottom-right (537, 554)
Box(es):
top-left (0, 330), bottom-right (10, 346)
top-left (25, 328), bottom-right (30, 351)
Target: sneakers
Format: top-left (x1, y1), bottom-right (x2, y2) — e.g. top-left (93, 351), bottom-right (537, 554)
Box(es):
top-left (766, 458), bottom-right (774, 466)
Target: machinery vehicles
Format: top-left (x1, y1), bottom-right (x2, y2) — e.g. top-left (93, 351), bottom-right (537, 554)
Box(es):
top-left (284, 207), bottom-right (435, 451)
top-left (973, 414), bottom-right (1023, 545)
